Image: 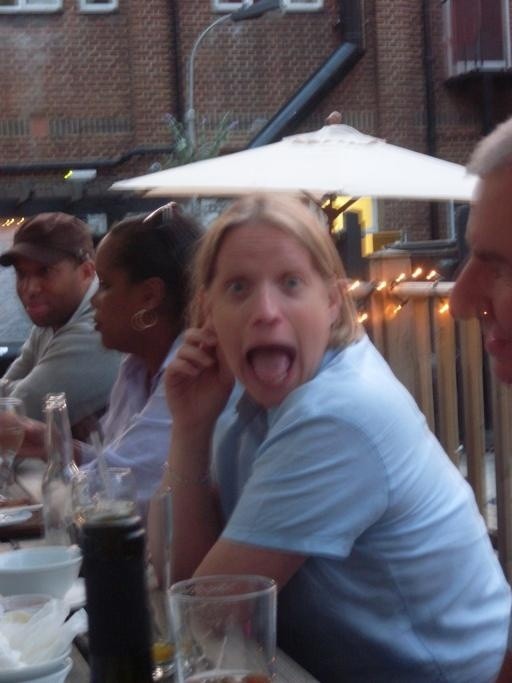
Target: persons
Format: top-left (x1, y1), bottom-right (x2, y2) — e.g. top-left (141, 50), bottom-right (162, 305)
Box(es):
top-left (1, 210), bottom-right (224, 525)
top-left (2, 209), bottom-right (113, 451)
top-left (447, 113), bottom-right (511, 682)
top-left (147, 187), bottom-right (511, 681)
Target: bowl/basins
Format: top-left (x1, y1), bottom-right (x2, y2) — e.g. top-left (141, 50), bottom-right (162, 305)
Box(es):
top-left (0, 640), bottom-right (76, 683)
top-left (0, 543), bottom-right (85, 601)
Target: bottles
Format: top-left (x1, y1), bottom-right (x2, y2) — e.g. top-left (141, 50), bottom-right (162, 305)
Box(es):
top-left (39, 390), bottom-right (85, 545)
top-left (83, 498), bottom-right (157, 681)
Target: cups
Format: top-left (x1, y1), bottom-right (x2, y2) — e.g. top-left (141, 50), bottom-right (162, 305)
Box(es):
top-left (70, 465), bottom-right (138, 523)
top-left (165, 572), bottom-right (280, 682)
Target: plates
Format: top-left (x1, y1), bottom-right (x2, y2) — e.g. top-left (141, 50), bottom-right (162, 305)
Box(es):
top-left (64, 575), bottom-right (90, 611)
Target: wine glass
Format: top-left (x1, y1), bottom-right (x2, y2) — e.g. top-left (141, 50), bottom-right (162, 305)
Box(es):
top-left (0, 396), bottom-right (27, 502)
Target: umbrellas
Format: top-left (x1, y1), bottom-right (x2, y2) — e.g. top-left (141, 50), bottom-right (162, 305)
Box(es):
top-left (105, 109), bottom-right (485, 203)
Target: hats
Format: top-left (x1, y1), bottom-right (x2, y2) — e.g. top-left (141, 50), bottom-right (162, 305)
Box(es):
top-left (0, 212), bottom-right (94, 266)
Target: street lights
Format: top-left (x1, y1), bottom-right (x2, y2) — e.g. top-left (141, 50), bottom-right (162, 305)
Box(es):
top-left (182, 0), bottom-right (292, 163)
